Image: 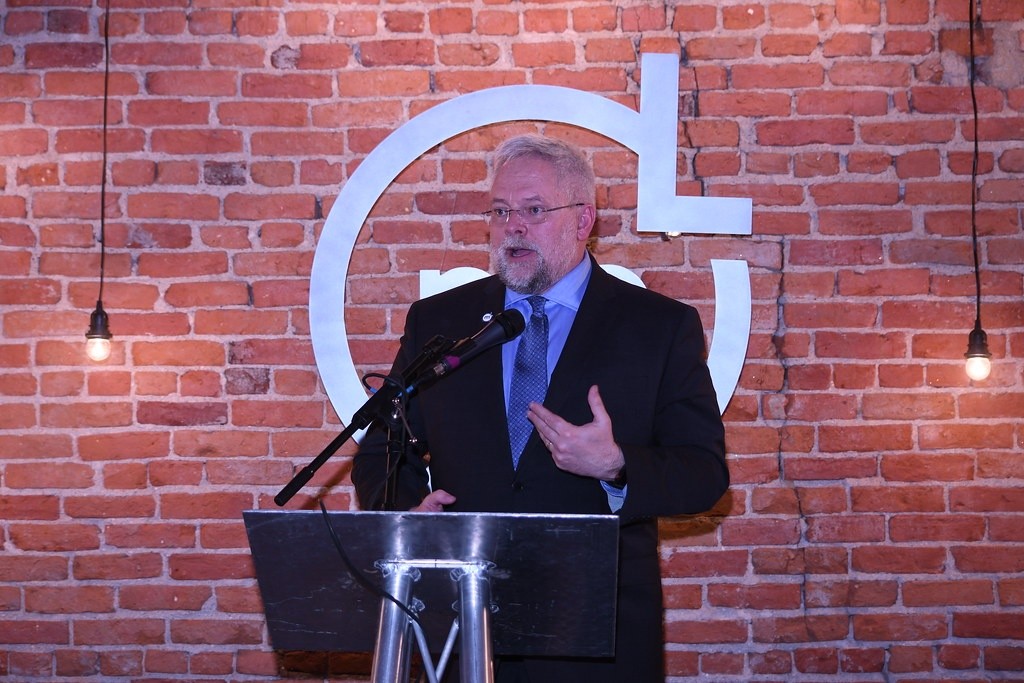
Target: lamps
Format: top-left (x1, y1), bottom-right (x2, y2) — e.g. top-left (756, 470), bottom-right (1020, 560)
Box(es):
top-left (962, 0), bottom-right (993, 383)
top-left (84, 0), bottom-right (114, 363)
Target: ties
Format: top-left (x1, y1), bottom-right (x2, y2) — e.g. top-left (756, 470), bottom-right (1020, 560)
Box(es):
top-left (507, 297), bottom-right (549, 470)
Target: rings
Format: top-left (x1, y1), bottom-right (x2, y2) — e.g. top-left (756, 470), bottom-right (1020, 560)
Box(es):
top-left (546, 441), bottom-right (552, 448)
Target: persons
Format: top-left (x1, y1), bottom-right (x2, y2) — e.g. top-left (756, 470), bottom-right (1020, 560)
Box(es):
top-left (351, 134), bottom-right (731, 683)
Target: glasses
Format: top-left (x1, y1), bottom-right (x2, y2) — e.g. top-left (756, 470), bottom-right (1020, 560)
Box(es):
top-left (483, 203), bottom-right (586, 225)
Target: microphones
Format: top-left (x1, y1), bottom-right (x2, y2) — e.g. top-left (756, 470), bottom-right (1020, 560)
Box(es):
top-left (410, 308), bottom-right (526, 399)
